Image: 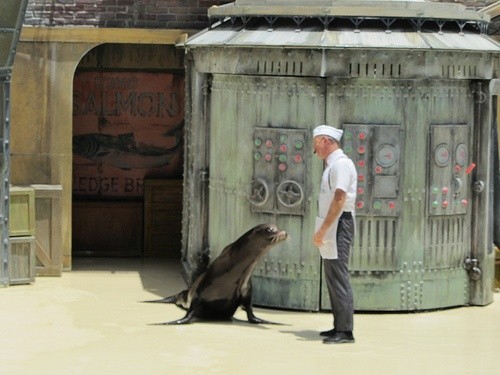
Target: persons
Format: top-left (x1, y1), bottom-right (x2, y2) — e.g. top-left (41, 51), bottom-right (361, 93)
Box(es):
top-left (312, 125), bottom-right (358, 344)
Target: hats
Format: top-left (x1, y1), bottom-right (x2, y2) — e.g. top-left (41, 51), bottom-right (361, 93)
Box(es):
top-left (313, 124), bottom-right (343, 142)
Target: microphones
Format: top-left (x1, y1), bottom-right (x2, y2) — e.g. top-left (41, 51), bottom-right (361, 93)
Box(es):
top-left (314, 138), bottom-right (323, 154)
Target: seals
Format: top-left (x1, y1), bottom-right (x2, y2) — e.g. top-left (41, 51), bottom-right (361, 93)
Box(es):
top-left (141, 222), bottom-right (292, 323)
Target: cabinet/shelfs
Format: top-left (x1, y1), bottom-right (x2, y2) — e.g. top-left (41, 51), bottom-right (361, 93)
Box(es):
top-left (71, 179), bottom-right (182, 257)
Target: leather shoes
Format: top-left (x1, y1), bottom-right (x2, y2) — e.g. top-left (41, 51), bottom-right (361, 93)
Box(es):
top-left (320, 327), bottom-right (336, 336)
top-left (323, 332), bottom-right (354, 343)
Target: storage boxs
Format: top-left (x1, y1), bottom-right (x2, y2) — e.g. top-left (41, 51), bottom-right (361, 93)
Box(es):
top-left (8, 184), bottom-right (63, 284)
